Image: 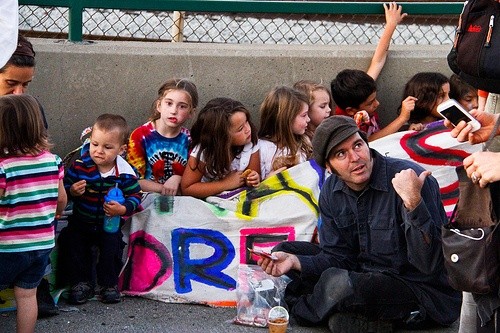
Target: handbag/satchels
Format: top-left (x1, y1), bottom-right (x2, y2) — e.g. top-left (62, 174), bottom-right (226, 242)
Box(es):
top-left (448, 0), bottom-right (500, 95)
top-left (440, 164), bottom-right (500, 293)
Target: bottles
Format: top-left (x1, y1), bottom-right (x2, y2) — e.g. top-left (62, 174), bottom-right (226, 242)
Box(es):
top-left (104, 188), bottom-right (125, 233)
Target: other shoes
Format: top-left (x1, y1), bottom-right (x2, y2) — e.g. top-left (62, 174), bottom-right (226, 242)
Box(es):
top-left (35, 278), bottom-right (57, 313)
top-left (328, 309), bottom-right (393, 333)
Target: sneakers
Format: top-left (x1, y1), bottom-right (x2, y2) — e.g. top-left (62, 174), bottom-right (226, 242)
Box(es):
top-left (71, 282), bottom-right (94, 301)
top-left (100, 284), bottom-right (120, 302)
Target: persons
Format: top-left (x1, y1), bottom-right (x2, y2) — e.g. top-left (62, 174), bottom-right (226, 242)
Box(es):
top-left (0, 35), bottom-right (37, 96)
top-left (59, 113), bottom-right (143, 304)
top-left (258, 85), bottom-right (315, 173)
top-left (330, 1), bottom-right (418, 142)
top-left (256, 115), bottom-right (463, 333)
top-left (126, 77), bottom-right (200, 196)
top-left (445, 109), bottom-right (500, 333)
top-left (293, 79), bottom-right (332, 159)
top-left (477, 89), bottom-right (500, 114)
top-left (395, 71), bottom-right (450, 131)
top-left (180, 96), bottom-right (261, 198)
top-left (447, 73), bottom-right (479, 118)
top-left (0, 94), bottom-right (68, 333)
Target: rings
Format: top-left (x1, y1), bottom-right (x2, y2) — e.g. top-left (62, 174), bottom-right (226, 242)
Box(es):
top-left (471, 172), bottom-right (480, 182)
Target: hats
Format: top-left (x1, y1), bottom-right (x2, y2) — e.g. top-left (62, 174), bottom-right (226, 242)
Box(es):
top-left (312, 115), bottom-right (358, 167)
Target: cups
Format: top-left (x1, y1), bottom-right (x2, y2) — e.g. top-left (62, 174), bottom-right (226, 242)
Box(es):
top-left (267, 318), bottom-right (288, 333)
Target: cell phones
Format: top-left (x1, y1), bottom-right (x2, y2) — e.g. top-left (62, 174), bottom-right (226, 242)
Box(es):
top-left (437, 99), bottom-right (481, 134)
